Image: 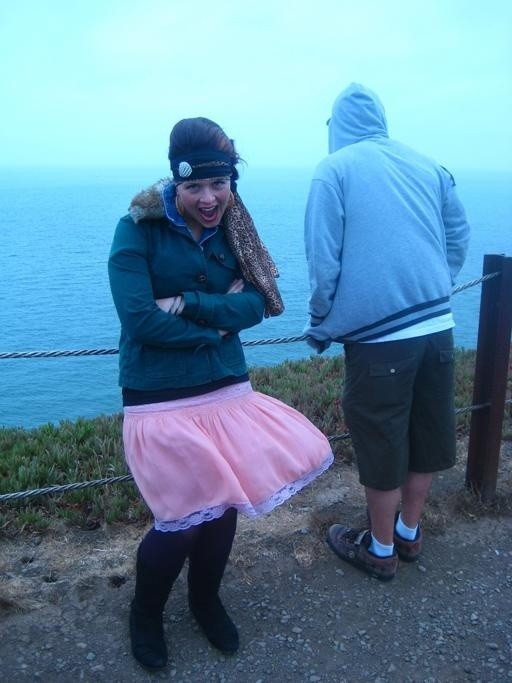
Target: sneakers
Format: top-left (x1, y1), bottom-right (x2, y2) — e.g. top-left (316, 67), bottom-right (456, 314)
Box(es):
top-left (366, 506), bottom-right (424, 563)
top-left (326, 522), bottom-right (400, 582)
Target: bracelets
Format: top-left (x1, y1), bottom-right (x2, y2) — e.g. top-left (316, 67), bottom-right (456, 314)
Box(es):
top-left (173, 294), bottom-right (185, 316)
top-left (167, 294), bottom-right (178, 313)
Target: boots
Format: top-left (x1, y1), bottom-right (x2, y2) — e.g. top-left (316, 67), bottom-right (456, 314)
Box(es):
top-left (128, 596), bottom-right (169, 673)
top-left (187, 591), bottom-right (240, 656)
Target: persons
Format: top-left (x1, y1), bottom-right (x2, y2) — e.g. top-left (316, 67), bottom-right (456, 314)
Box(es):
top-left (301, 80), bottom-right (473, 582)
top-left (108, 115), bottom-right (336, 672)
